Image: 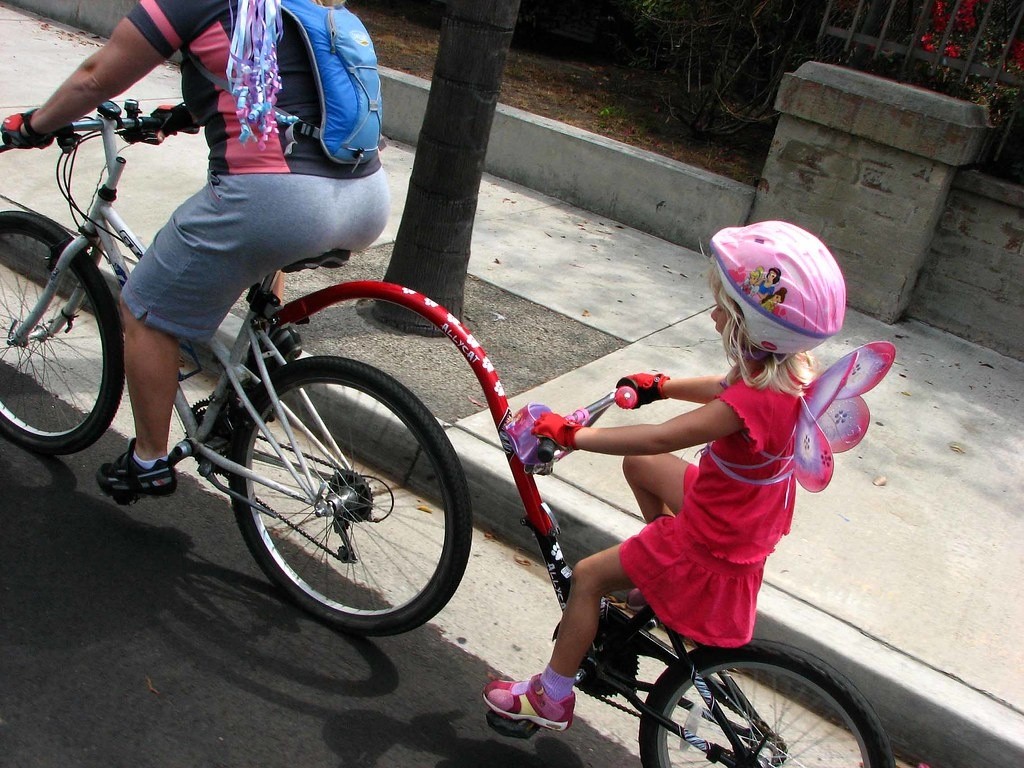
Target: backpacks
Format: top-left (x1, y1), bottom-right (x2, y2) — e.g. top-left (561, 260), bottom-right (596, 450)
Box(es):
top-left (186, 0), bottom-right (382, 175)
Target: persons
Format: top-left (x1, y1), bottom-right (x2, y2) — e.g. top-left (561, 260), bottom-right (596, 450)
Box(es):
top-left (479, 221), bottom-right (894, 729)
top-left (0, 0), bottom-right (391, 497)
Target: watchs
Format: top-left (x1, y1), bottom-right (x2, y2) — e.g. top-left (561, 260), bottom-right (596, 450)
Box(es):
top-left (21, 107), bottom-right (51, 137)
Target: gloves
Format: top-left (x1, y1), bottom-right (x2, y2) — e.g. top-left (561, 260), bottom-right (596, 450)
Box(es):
top-left (530, 413), bottom-right (585, 451)
top-left (624, 373), bottom-right (671, 410)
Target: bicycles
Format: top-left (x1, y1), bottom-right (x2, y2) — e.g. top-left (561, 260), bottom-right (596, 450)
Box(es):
top-left (1, 95), bottom-right (896, 768)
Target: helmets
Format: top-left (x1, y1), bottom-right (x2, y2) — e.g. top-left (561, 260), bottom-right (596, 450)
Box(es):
top-left (709, 221), bottom-right (846, 352)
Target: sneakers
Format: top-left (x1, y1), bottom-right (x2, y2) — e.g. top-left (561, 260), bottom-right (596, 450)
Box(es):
top-left (245, 325), bottom-right (303, 380)
top-left (626, 587), bottom-right (645, 611)
top-left (96, 439), bottom-right (177, 496)
top-left (481, 674), bottom-right (576, 730)
